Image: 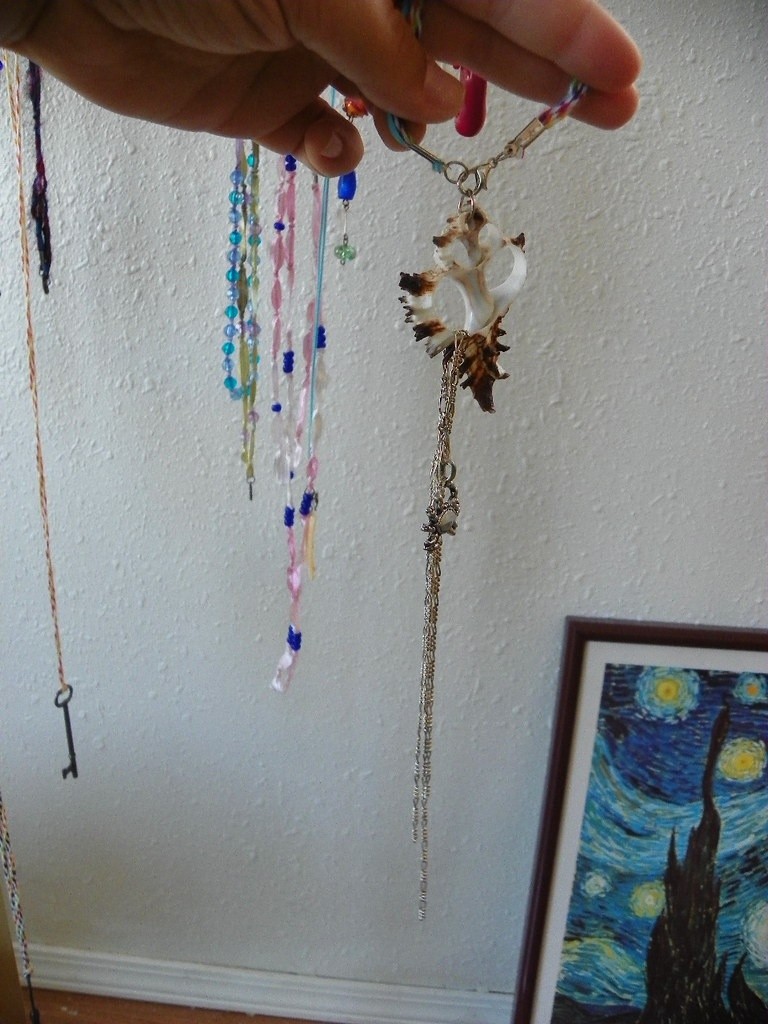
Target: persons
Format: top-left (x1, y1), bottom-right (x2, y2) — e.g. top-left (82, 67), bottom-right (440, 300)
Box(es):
top-left (0, 0), bottom-right (641, 176)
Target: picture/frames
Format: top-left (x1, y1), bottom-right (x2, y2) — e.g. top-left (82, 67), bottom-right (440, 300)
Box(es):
top-left (508, 613), bottom-right (768, 1024)
